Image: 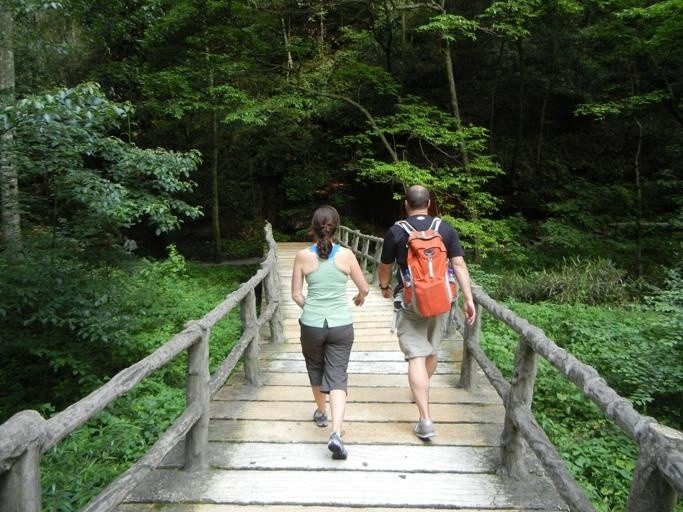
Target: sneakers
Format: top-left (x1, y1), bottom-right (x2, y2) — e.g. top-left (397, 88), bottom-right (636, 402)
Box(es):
top-left (414, 419), bottom-right (436, 439)
top-left (313, 406), bottom-right (330, 428)
top-left (326, 430), bottom-right (348, 461)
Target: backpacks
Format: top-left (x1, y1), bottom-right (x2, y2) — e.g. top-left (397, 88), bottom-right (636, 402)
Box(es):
top-left (395, 216), bottom-right (459, 319)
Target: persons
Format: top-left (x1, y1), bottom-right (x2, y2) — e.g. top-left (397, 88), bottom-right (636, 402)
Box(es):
top-left (290, 204), bottom-right (369, 461)
top-left (377, 186), bottom-right (475, 439)
top-left (124, 234), bottom-right (138, 253)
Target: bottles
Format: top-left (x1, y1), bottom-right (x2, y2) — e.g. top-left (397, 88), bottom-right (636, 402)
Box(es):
top-left (403, 268), bottom-right (410, 284)
top-left (448, 269), bottom-right (455, 282)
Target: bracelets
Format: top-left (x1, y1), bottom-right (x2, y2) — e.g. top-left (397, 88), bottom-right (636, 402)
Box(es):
top-left (379, 283), bottom-right (390, 291)
